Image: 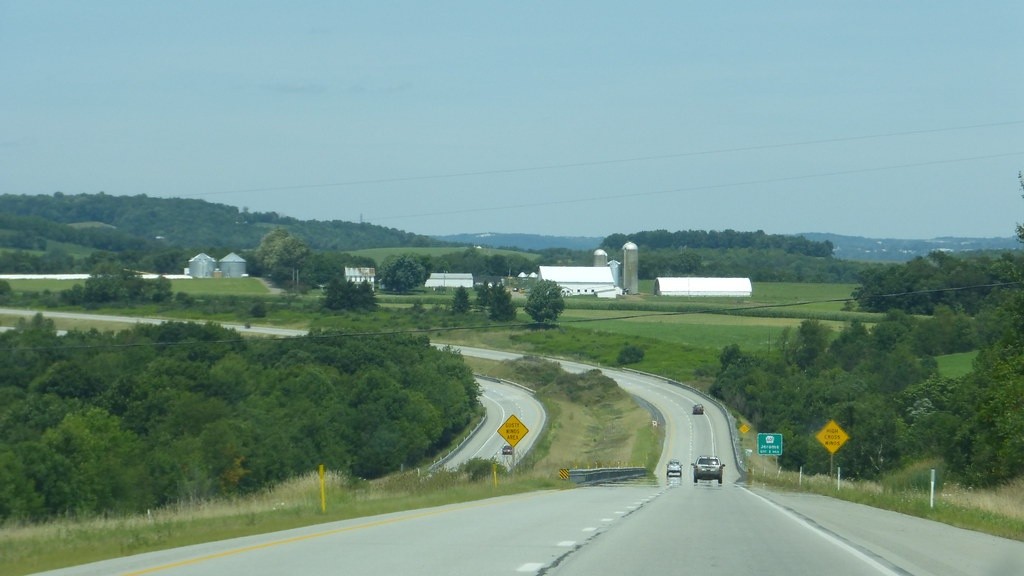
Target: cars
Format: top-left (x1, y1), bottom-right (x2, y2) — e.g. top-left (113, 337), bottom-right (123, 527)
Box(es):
top-left (693, 404), bottom-right (705, 416)
top-left (502, 446), bottom-right (512, 454)
top-left (666, 460), bottom-right (683, 476)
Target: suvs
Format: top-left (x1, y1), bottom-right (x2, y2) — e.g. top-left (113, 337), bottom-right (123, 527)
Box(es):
top-left (692, 456), bottom-right (726, 486)
